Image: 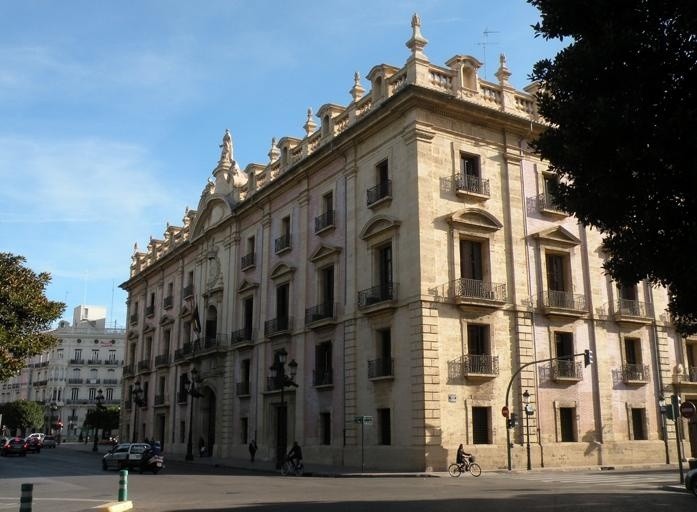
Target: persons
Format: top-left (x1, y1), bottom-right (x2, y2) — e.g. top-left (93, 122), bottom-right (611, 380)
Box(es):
top-left (198, 436), bottom-right (205, 457)
top-left (288, 441), bottom-right (302, 468)
top-left (249, 439), bottom-right (257, 462)
top-left (456, 443), bottom-right (471, 472)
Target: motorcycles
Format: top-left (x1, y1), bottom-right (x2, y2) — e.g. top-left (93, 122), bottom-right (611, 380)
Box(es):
top-left (143, 446), bottom-right (164, 475)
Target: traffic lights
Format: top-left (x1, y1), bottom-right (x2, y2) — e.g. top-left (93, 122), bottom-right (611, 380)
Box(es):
top-left (511, 413), bottom-right (518, 427)
top-left (585, 350), bottom-right (594, 368)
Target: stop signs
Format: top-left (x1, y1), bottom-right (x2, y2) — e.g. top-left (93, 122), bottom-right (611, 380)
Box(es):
top-left (502, 407), bottom-right (509, 417)
top-left (680, 401), bottom-right (696, 418)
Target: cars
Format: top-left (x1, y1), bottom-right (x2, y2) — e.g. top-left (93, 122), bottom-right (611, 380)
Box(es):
top-left (0, 433), bottom-right (56, 457)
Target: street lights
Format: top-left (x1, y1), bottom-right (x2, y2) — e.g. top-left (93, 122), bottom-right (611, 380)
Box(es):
top-left (93, 389), bottom-right (104, 451)
top-left (523, 390), bottom-right (533, 470)
top-left (268, 348), bottom-right (297, 464)
top-left (659, 395), bottom-right (669, 464)
top-left (185, 368), bottom-right (203, 461)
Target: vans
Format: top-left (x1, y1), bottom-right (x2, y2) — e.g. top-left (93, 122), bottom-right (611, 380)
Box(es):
top-left (102, 443), bottom-right (151, 471)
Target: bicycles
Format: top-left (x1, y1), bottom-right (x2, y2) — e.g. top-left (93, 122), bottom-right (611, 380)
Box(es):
top-left (448, 455), bottom-right (481, 477)
top-left (281, 455), bottom-right (304, 477)
